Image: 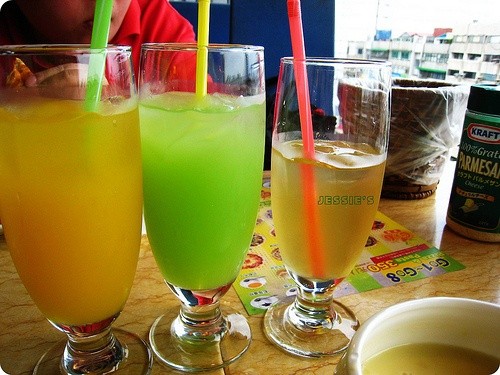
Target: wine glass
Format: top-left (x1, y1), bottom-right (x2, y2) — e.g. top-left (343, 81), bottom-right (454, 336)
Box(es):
top-left (270, 56), bottom-right (392, 357)
top-left (0, 44), bottom-right (155, 375)
top-left (137, 43), bottom-right (266, 372)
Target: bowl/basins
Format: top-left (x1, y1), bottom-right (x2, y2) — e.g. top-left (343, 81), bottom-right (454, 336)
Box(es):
top-left (347, 298), bottom-right (500, 375)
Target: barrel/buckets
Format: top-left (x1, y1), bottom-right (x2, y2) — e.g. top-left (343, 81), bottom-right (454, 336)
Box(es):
top-left (337, 77), bottom-right (471, 199)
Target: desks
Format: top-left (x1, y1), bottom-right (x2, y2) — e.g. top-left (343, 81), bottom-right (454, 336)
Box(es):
top-left (0, 170), bottom-right (500, 375)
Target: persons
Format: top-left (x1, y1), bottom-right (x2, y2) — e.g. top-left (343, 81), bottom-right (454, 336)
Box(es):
top-left (0, 0), bottom-right (218, 102)
